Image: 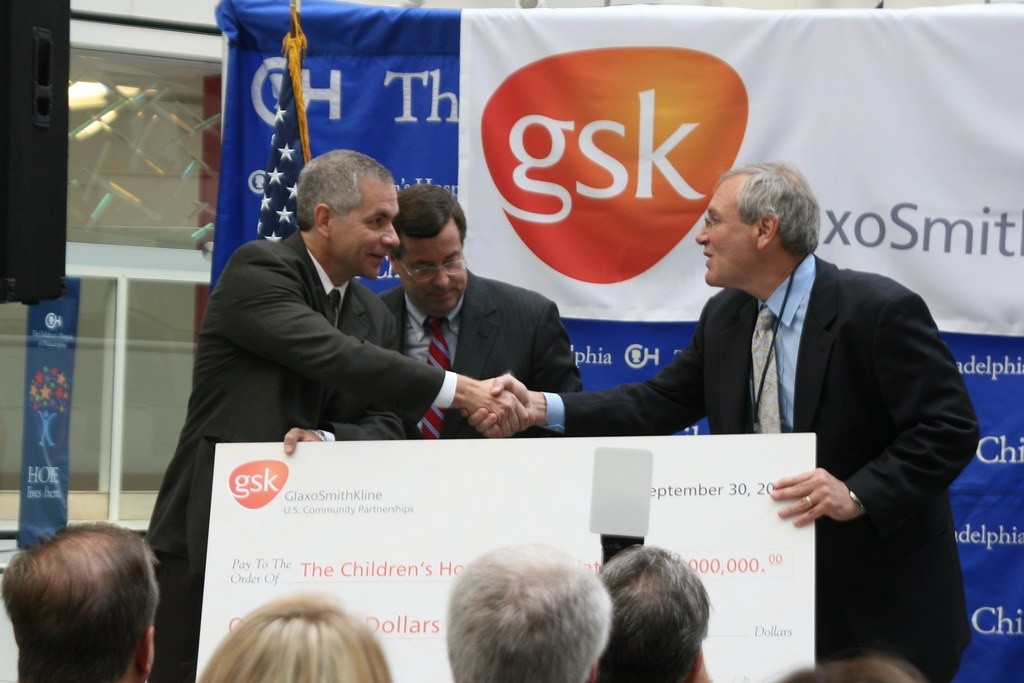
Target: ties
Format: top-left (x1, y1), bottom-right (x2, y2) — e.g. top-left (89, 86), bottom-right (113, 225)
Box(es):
top-left (419, 315), bottom-right (451, 439)
top-left (751, 307), bottom-right (783, 433)
top-left (326, 288), bottom-right (341, 328)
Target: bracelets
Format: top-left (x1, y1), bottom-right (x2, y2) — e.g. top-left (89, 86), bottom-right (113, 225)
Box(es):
top-left (850, 491), bottom-right (867, 515)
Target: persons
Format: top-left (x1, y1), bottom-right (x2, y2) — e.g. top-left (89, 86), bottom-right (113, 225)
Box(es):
top-left (372, 184), bottom-right (580, 440)
top-left (0, 523), bottom-right (922, 682)
top-left (143, 148), bottom-right (531, 683)
top-left (464, 162), bottom-right (976, 682)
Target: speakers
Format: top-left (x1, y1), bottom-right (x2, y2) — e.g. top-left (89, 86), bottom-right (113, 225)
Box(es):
top-left (0, 0), bottom-right (70, 305)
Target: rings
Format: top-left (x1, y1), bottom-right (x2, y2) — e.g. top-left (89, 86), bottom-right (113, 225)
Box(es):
top-left (807, 497), bottom-right (813, 507)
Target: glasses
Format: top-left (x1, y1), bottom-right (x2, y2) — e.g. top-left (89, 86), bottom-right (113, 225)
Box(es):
top-left (703, 213), bottom-right (745, 232)
top-left (397, 257), bottom-right (465, 282)
